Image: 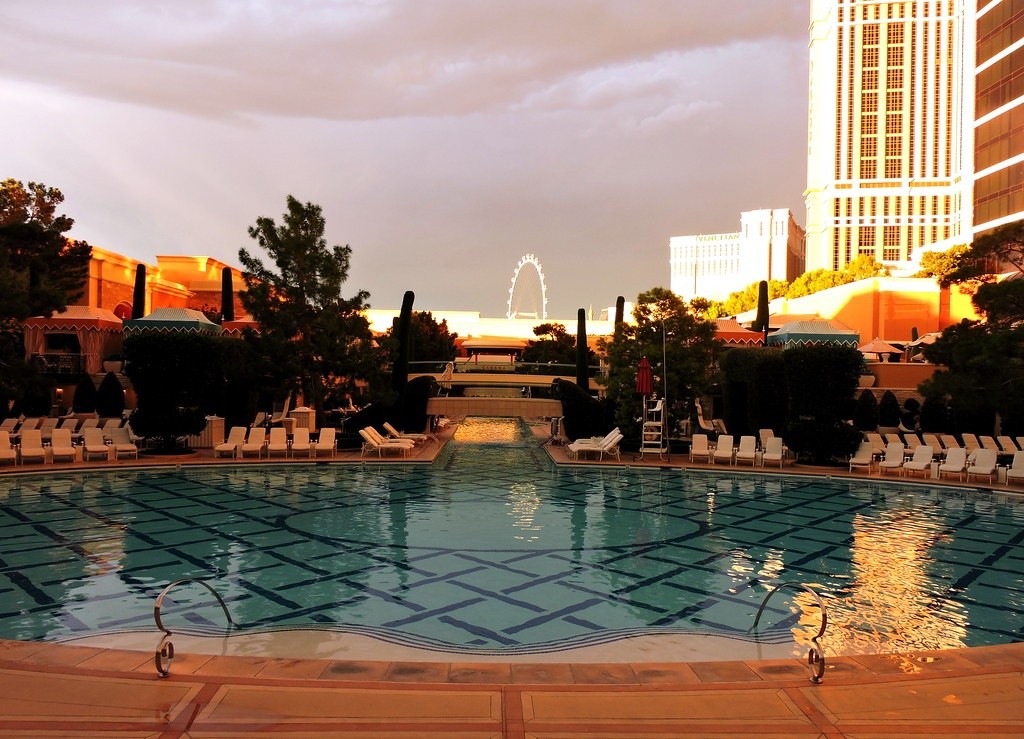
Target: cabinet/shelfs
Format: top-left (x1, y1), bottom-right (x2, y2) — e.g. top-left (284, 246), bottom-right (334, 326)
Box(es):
top-left (632, 395), bottom-right (669, 462)
top-left (188, 414), bottom-right (225, 448)
top-left (289, 408), bottom-right (315, 431)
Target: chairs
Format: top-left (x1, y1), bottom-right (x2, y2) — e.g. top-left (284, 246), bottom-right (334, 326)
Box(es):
top-left (570, 427), bottom-right (624, 461)
top-left (885, 434), bottom-right (914, 454)
top-left (967, 449), bottom-right (999, 485)
top-left (903, 445), bottom-right (934, 479)
top-left (998, 436), bottom-right (1024, 486)
top-left (941, 435), bottom-right (960, 449)
top-left (923, 435), bottom-right (942, 454)
top-left (979, 436), bottom-right (998, 449)
top-left (962, 433), bottom-right (981, 454)
top-left (759, 429), bottom-right (774, 449)
top-left (359, 418), bottom-right (451, 458)
top-left (0, 400), bottom-right (338, 467)
top-left (849, 434), bottom-right (885, 475)
top-left (761, 437), bottom-right (784, 469)
top-left (938, 447), bottom-right (967, 482)
top-left (904, 434), bottom-right (922, 449)
top-left (735, 436), bottom-right (755, 467)
top-left (878, 443), bottom-right (904, 476)
top-left (691, 434), bottom-right (710, 463)
top-left (712, 435), bottom-right (733, 465)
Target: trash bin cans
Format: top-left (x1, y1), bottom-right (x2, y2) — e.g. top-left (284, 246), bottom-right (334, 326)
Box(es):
top-left (930, 463), bottom-right (940, 479)
top-left (998, 466), bottom-right (1007, 485)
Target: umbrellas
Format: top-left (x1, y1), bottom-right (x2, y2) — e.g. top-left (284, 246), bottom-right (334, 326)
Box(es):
top-left (857, 337), bottom-right (904, 362)
top-left (636, 356), bottom-right (653, 461)
top-left (904, 331), bottom-right (942, 348)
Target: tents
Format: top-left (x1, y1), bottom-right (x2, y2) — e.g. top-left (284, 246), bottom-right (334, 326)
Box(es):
top-left (22, 305), bottom-right (261, 372)
top-left (709, 319), bottom-right (860, 352)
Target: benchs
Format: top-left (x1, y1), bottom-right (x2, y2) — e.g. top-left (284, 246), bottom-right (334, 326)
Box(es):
top-left (695, 398), bottom-right (728, 434)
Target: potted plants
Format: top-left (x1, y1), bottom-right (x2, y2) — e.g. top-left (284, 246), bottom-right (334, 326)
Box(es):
top-left (856, 388), bottom-right (877, 442)
top-left (72, 372), bottom-right (98, 423)
top-left (98, 371), bottom-right (125, 426)
top-left (104, 354), bottom-right (123, 373)
top-left (859, 358), bottom-right (876, 388)
top-left (878, 390), bottom-right (900, 434)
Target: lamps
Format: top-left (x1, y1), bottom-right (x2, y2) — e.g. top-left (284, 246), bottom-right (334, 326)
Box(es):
top-left (56, 388), bottom-right (63, 405)
